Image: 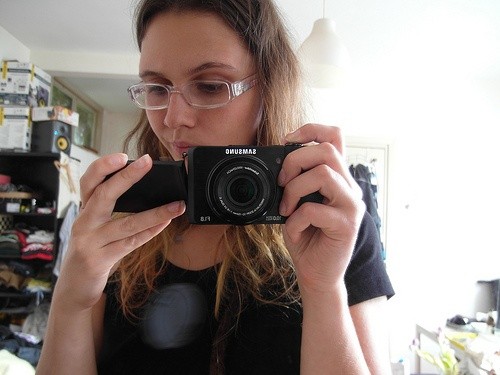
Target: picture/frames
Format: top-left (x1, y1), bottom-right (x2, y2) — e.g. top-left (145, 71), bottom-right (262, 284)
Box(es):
top-left (343, 141), bottom-right (389, 268)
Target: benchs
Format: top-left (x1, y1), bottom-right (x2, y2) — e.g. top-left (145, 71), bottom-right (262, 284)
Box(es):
top-left (409, 325), bottom-right (489, 375)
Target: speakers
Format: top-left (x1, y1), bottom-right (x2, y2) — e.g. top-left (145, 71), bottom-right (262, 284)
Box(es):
top-left (30, 120), bottom-right (72, 155)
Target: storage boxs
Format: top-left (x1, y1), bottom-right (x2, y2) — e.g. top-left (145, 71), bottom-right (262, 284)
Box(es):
top-left (0, 58), bottom-right (81, 126)
top-left (0, 106), bottom-right (35, 151)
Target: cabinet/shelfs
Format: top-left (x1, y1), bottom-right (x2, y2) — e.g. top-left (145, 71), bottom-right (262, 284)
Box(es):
top-left (0, 151), bottom-right (82, 362)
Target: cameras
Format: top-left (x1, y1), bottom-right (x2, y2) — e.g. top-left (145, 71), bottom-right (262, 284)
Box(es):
top-left (188, 144), bottom-right (325, 226)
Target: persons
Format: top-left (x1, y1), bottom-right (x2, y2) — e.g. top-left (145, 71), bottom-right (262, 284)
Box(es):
top-left (33, 0), bottom-right (397, 374)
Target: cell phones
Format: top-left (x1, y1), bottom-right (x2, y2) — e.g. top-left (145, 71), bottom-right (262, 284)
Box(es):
top-left (102, 159), bottom-right (188, 221)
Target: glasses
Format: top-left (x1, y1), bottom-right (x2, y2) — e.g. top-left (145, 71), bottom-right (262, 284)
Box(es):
top-left (127, 71), bottom-right (260, 111)
